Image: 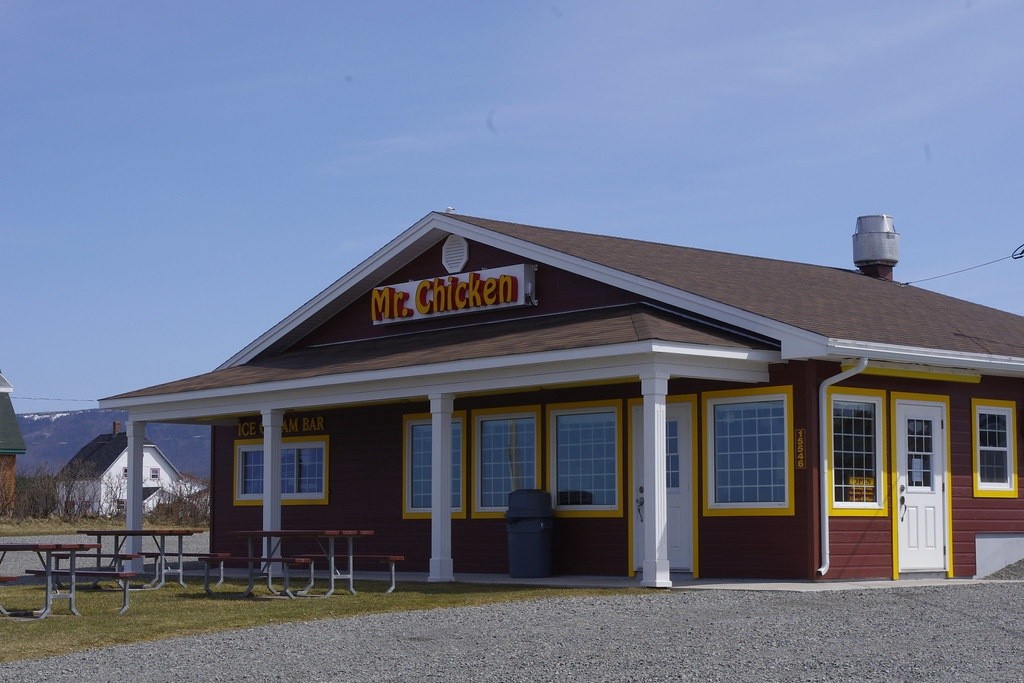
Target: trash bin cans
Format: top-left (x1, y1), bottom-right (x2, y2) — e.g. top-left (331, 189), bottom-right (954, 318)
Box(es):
top-left (504, 489), bottom-right (553, 578)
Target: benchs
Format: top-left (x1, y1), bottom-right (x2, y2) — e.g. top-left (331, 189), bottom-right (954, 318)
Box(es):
top-left (25, 569), bottom-right (138, 615)
top-left (51, 553), bottom-right (141, 590)
top-left (197, 556), bottom-right (312, 601)
top-left (289, 553), bottom-right (407, 594)
top-left (136, 552), bottom-right (231, 591)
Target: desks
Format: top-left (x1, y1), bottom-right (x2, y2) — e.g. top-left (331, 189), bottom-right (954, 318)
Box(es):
top-left (225, 529), bottom-right (375, 598)
top-left (76, 529), bottom-right (204, 590)
top-left (0, 543), bottom-right (103, 619)
